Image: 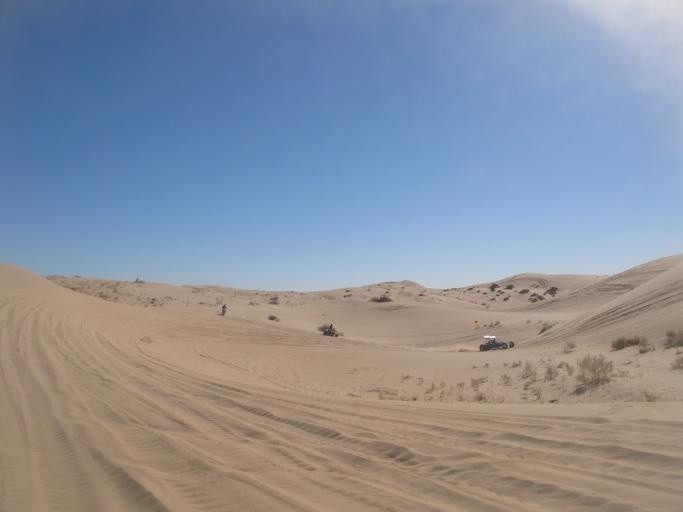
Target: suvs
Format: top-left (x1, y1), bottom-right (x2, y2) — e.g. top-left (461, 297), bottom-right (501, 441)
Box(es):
top-left (323, 328), bottom-right (339, 338)
top-left (479, 335), bottom-right (515, 352)
